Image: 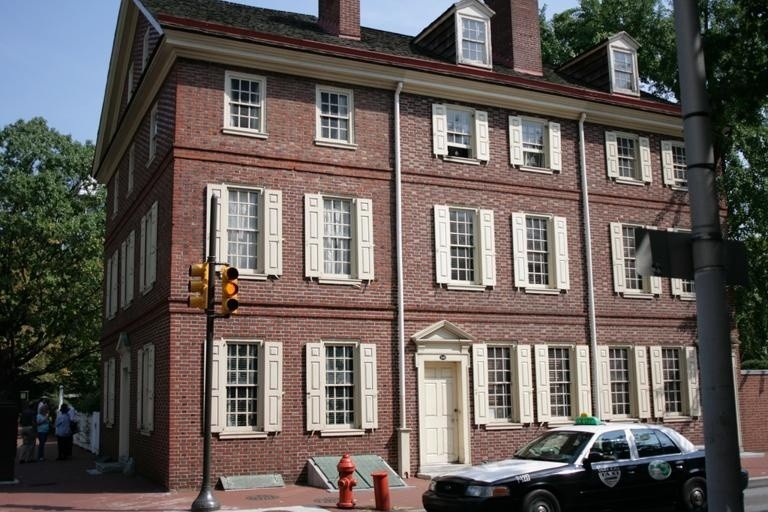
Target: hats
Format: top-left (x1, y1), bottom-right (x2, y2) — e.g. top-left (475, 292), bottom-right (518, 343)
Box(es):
top-left (59, 403), bottom-right (71, 412)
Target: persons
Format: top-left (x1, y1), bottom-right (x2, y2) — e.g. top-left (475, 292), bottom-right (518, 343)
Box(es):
top-left (54, 403), bottom-right (74, 463)
top-left (60, 403), bottom-right (76, 461)
top-left (18, 398), bottom-right (38, 464)
top-left (600, 438), bottom-right (617, 461)
top-left (35, 403), bottom-right (50, 463)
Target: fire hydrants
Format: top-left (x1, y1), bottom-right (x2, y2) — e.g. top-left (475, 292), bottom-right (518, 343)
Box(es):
top-left (337, 453), bottom-right (358, 509)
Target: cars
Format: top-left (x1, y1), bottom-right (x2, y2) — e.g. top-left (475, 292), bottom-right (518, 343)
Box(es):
top-left (423, 412), bottom-right (748, 512)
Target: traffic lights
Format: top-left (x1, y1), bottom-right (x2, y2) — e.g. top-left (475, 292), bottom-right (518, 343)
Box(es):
top-left (222, 267), bottom-right (239, 316)
top-left (188, 263), bottom-right (208, 310)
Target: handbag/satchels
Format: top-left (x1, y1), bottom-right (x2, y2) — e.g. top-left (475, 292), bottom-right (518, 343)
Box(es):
top-left (69, 419), bottom-right (79, 434)
